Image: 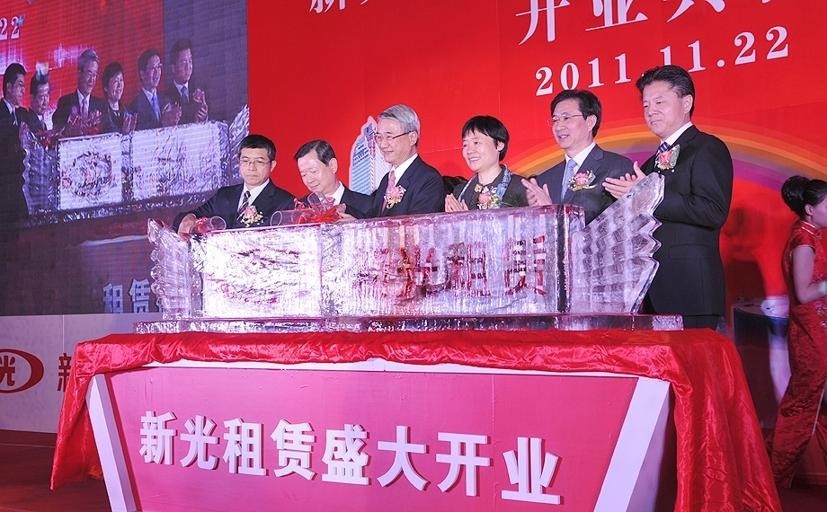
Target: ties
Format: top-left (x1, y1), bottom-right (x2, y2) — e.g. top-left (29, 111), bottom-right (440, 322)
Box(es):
top-left (151, 92), bottom-right (160, 122)
top-left (382, 171), bottom-right (395, 216)
top-left (180, 86), bottom-right (188, 104)
top-left (235, 191), bottom-right (251, 228)
top-left (561, 160), bottom-right (577, 203)
top-left (654, 141), bottom-right (671, 168)
top-left (81, 99), bottom-right (88, 135)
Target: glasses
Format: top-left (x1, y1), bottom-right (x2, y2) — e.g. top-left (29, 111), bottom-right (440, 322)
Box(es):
top-left (548, 114), bottom-right (588, 126)
top-left (238, 157), bottom-right (270, 169)
top-left (146, 64), bottom-right (164, 71)
top-left (374, 130), bottom-right (411, 143)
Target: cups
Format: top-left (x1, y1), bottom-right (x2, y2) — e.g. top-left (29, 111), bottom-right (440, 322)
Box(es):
top-left (307, 192), bottom-right (331, 213)
top-left (190, 216), bottom-right (226, 234)
top-left (270, 209), bottom-right (300, 227)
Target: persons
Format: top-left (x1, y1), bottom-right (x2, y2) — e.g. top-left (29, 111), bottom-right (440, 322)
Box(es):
top-left (289, 139), bottom-right (371, 218)
top-left (372, 102), bottom-right (443, 215)
top-left (601, 63), bottom-right (734, 330)
top-left (520, 87), bottom-right (635, 226)
top-left (442, 115), bottom-right (527, 212)
top-left (772, 175), bottom-right (827, 490)
top-left (173, 135), bottom-right (297, 233)
top-left (1, 36), bottom-right (207, 218)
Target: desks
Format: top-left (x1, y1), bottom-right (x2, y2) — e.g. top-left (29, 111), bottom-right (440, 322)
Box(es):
top-left (77, 323), bottom-right (752, 511)
